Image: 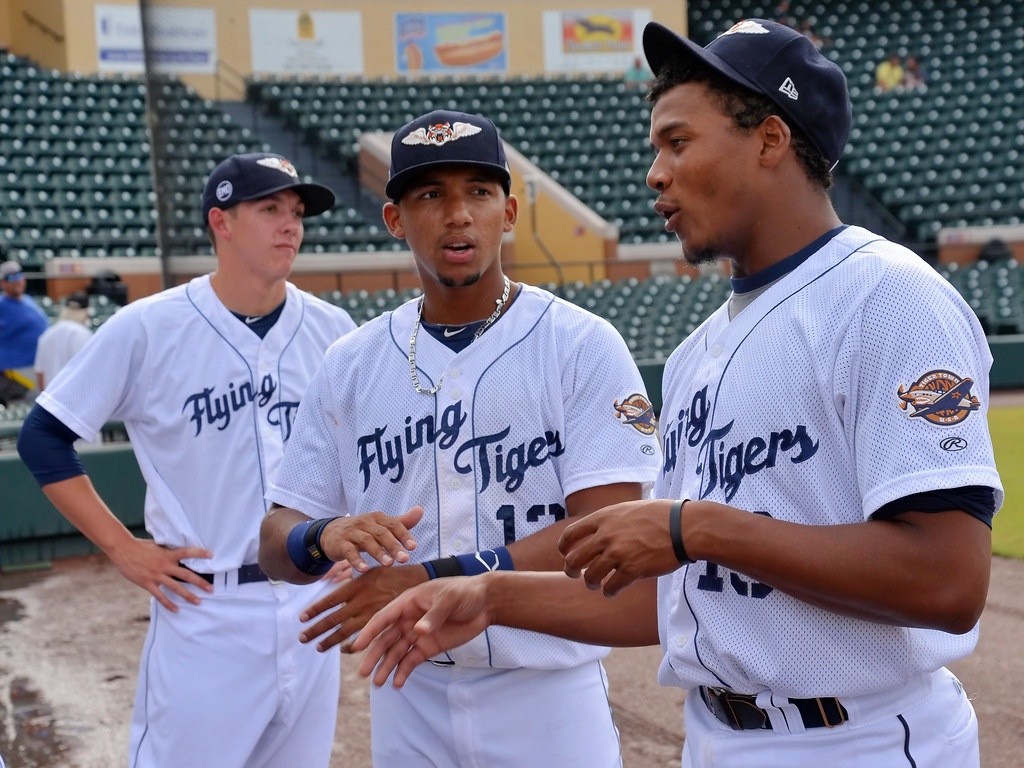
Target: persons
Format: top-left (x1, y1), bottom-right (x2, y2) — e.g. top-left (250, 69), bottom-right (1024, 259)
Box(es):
top-left (778, 15), bottom-right (825, 51)
top-left (259, 109), bottom-right (662, 766)
top-left (874, 53), bottom-right (925, 96)
top-left (343, 16), bottom-right (1006, 766)
top-left (13, 152), bottom-right (361, 768)
top-left (0, 260), bottom-right (98, 405)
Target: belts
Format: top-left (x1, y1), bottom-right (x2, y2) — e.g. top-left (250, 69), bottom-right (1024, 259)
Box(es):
top-left (173, 564), bottom-right (280, 587)
top-left (696, 683), bottom-right (846, 730)
top-left (425, 658), bottom-right (454, 669)
top-left (431, 660), bottom-right (455, 666)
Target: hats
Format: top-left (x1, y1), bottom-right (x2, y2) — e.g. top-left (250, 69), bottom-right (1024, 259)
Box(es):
top-left (202, 152), bottom-right (337, 217)
top-left (642, 19), bottom-right (852, 169)
top-left (0, 261), bottom-right (21, 280)
top-left (66, 291), bottom-right (90, 309)
top-left (386, 109), bottom-right (508, 198)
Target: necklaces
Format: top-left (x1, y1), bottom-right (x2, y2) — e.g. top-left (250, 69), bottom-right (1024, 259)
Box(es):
top-left (407, 273), bottom-right (511, 394)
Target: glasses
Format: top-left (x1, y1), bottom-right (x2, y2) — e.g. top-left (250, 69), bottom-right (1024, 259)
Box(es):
top-left (5, 273), bottom-right (24, 283)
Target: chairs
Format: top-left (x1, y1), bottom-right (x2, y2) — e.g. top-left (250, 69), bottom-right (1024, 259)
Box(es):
top-left (0, 0), bottom-right (1024, 434)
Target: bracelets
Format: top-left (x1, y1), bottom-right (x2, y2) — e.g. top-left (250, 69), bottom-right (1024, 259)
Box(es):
top-left (669, 498), bottom-right (698, 567)
